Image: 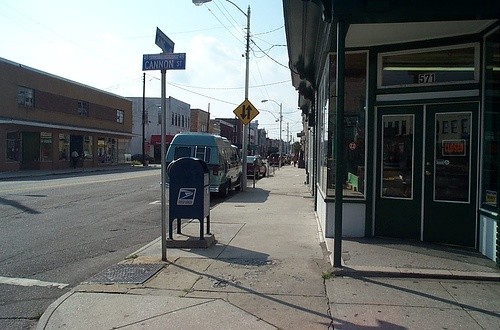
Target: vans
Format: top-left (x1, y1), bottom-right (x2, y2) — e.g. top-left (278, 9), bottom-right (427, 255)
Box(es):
top-left (158, 132), bottom-right (243, 200)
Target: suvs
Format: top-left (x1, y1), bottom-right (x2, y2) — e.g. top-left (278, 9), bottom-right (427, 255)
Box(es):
top-left (130, 153), bottom-right (156, 165)
top-left (266, 153), bottom-right (291, 167)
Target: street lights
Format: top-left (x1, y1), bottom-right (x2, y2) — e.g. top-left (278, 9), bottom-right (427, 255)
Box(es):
top-left (261, 99), bottom-right (282, 170)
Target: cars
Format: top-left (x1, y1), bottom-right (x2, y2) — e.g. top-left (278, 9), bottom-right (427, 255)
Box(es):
top-left (246, 155), bottom-right (267, 180)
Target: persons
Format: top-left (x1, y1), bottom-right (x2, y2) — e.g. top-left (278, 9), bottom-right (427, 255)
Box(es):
top-left (71, 150), bottom-right (79, 168)
top-left (292, 156), bottom-right (296, 167)
